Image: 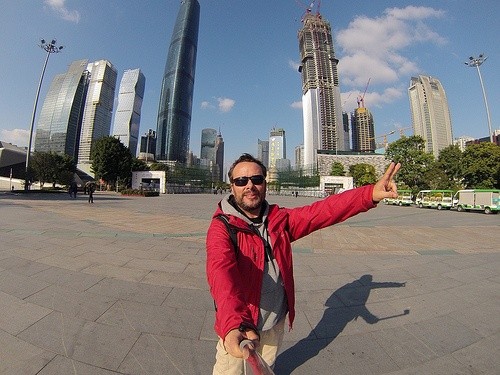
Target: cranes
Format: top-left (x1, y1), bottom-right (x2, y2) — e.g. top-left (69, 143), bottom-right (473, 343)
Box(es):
top-left (366, 129), bottom-right (396, 143)
top-left (400, 126), bottom-right (413, 134)
top-left (357, 77), bottom-right (371, 108)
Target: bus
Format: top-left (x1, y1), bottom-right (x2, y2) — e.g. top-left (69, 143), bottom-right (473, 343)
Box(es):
top-left (452, 189), bottom-right (500, 214)
top-left (415, 189), bottom-right (455, 210)
top-left (383, 189), bottom-right (414, 207)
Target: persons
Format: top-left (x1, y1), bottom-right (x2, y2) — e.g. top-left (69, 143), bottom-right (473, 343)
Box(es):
top-left (206, 154), bottom-right (401, 375)
top-left (84, 183), bottom-right (95, 203)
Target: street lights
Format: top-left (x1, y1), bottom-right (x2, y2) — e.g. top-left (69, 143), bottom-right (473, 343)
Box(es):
top-left (465, 53), bottom-right (493, 143)
top-left (145, 129), bottom-right (155, 164)
top-left (24, 39), bottom-right (64, 191)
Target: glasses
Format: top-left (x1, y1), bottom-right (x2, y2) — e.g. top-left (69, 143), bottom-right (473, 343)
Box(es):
top-left (232, 175), bottom-right (266, 186)
